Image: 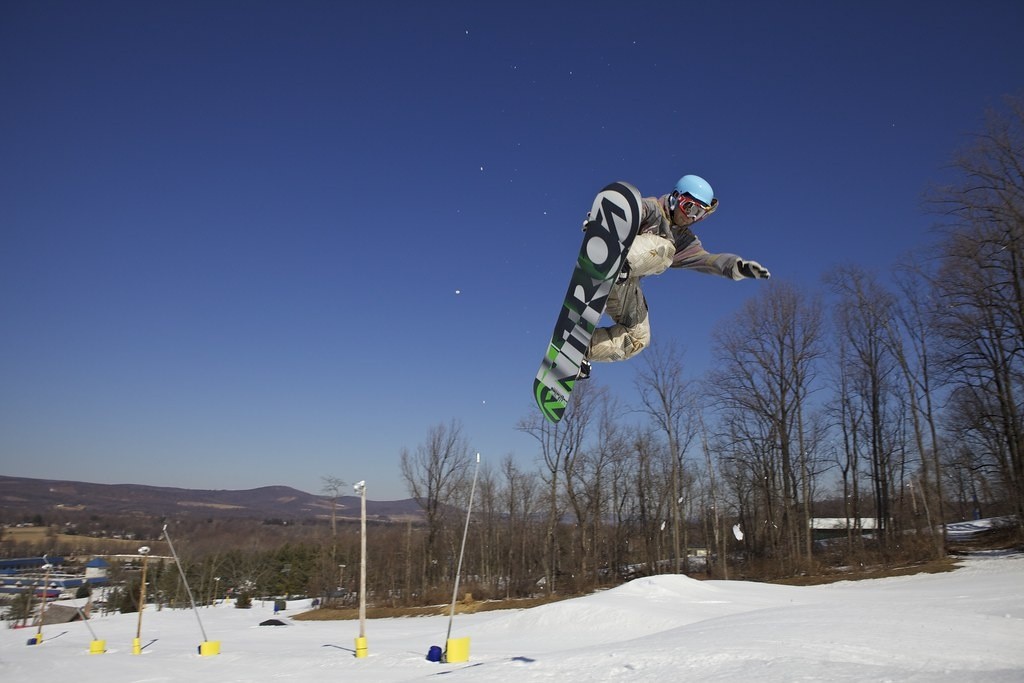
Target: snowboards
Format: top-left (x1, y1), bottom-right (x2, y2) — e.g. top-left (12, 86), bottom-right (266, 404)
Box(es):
top-left (532, 181), bottom-right (644, 425)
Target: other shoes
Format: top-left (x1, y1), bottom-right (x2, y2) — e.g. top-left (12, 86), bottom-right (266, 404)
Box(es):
top-left (616, 257), bottom-right (630, 285)
top-left (580, 360), bottom-right (591, 379)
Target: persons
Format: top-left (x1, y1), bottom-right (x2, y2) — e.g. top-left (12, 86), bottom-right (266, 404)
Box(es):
top-left (578, 175), bottom-right (771, 380)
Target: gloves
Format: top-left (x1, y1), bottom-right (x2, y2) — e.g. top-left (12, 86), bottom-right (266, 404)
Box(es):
top-left (733, 259), bottom-right (770, 280)
top-left (581, 211), bottom-right (592, 232)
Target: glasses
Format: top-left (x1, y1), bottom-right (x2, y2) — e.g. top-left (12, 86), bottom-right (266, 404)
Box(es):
top-left (679, 198), bottom-right (708, 222)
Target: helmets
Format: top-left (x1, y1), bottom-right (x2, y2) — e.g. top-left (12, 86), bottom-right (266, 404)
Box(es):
top-left (674, 174), bottom-right (714, 206)
